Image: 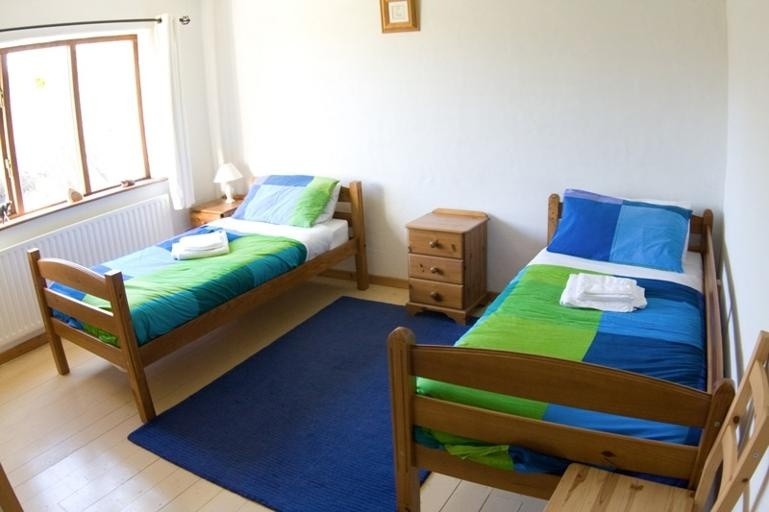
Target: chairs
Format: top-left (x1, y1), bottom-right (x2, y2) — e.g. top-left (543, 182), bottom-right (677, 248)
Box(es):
top-left (543, 331), bottom-right (769, 512)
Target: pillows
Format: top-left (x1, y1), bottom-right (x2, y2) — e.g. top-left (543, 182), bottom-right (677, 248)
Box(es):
top-left (546, 188), bottom-right (693, 273)
top-left (232, 175), bottom-right (340, 228)
top-left (623, 198), bottom-right (692, 261)
top-left (315, 182), bottom-right (341, 224)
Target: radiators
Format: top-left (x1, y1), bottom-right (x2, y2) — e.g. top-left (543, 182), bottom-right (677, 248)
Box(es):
top-left (0, 194), bottom-right (174, 355)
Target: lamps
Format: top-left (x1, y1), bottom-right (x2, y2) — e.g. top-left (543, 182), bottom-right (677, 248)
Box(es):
top-left (213, 163), bottom-right (242, 204)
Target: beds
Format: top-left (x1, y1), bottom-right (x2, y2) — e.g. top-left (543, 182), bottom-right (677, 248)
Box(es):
top-left (27, 176), bottom-right (369, 423)
top-left (386, 193), bottom-right (736, 512)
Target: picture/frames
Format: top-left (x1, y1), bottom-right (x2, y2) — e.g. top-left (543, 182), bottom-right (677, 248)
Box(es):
top-left (379, 0), bottom-right (421, 33)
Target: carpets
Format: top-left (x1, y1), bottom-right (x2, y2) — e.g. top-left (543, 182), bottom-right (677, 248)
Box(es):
top-left (128, 295), bottom-right (479, 512)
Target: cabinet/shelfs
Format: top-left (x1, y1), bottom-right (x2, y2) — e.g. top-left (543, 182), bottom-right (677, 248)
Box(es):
top-left (406, 208), bottom-right (490, 326)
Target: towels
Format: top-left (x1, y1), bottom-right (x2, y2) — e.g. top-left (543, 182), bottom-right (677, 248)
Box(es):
top-left (172, 230), bottom-right (229, 260)
top-left (560, 271), bottom-right (647, 312)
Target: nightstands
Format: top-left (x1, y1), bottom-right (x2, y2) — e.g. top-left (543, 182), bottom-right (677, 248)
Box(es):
top-left (191, 195), bottom-right (244, 228)
